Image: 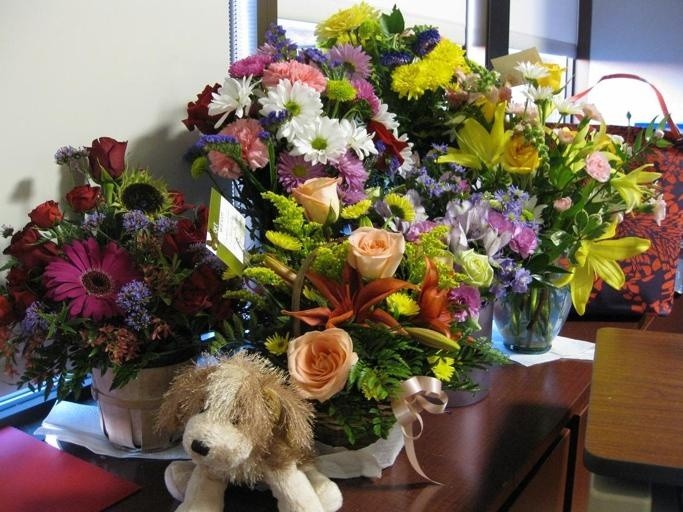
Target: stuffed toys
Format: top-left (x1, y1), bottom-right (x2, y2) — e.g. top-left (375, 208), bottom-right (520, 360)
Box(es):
top-left (153, 347), bottom-right (343, 511)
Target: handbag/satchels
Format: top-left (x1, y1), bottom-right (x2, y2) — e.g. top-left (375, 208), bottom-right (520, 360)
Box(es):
top-left (544, 73), bottom-right (683, 317)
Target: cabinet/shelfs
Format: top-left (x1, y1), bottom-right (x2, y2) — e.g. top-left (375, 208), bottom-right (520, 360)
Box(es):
top-left (0, 321), bottom-right (640, 512)
